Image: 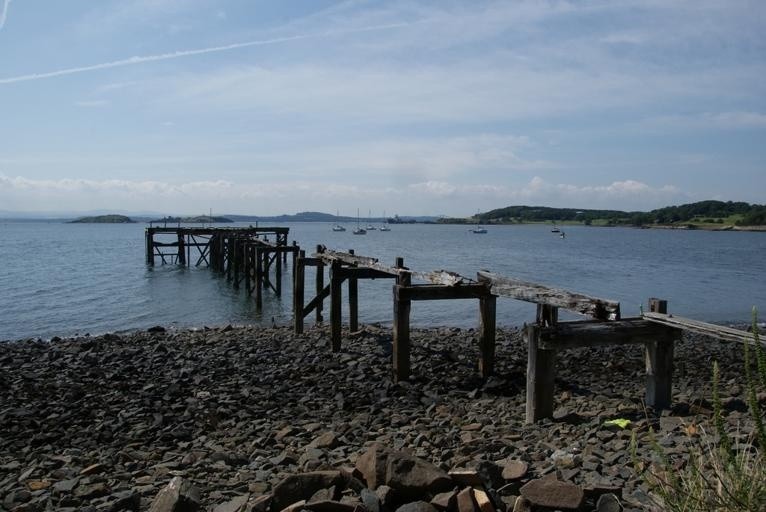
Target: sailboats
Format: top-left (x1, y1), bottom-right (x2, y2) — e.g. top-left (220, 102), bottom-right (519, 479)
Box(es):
top-left (353, 207), bottom-right (365, 235)
top-left (366, 207), bottom-right (375, 230)
top-left (333, 208), bottom-right (347, 232)
top-left (474, 206), bottom-right (489, 235)
top-left (379, 209), bottom-right (390, 231)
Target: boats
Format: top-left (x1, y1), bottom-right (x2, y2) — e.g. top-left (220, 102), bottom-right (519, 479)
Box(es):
top-left (559, 232), bottom-right (566, 240)
top-left (551, 224), bottom-right (561, 233)
